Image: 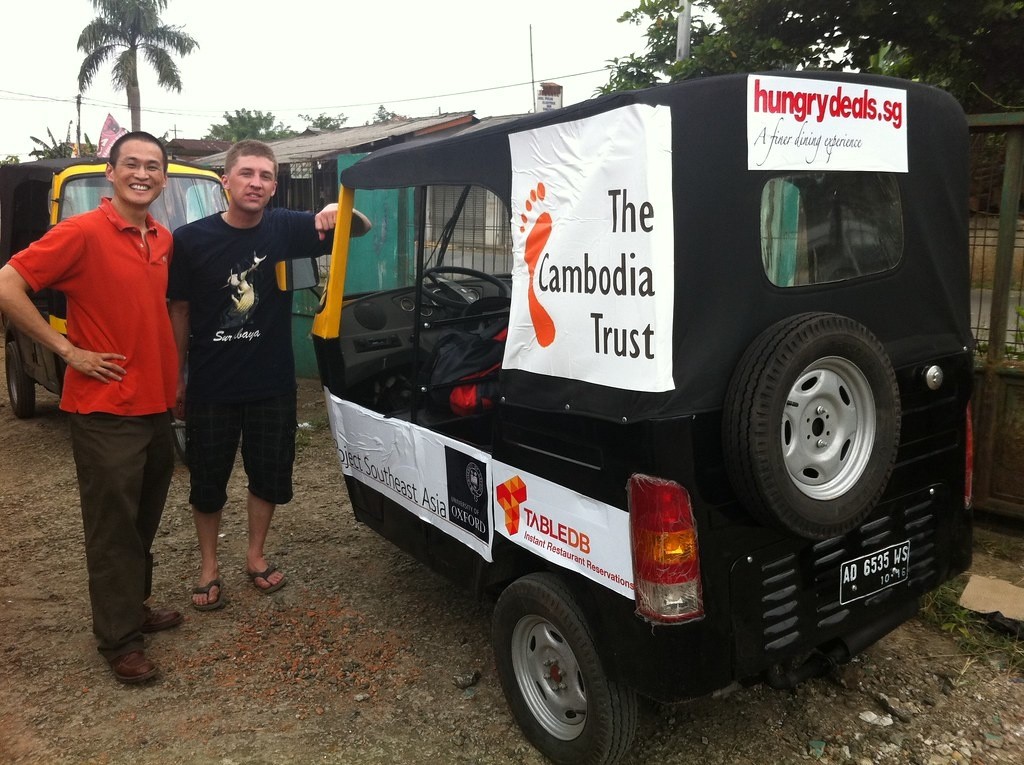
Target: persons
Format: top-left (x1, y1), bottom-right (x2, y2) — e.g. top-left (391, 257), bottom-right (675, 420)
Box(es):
top-left (0, 132), bottom-right (182, 683)
top-left (166, 141), bottom-right (370, 612)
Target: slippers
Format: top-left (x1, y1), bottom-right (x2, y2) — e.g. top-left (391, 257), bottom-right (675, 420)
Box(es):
top-left (250, 563), bottom-right (288, 595)
top-left (192, 575), bottom-right (223, 610)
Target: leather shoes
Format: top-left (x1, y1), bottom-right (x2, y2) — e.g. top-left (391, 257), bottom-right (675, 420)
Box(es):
top-left (139, 604), bottom-right (184, 632)
top-left (111, 649), bottom-right (157, 683)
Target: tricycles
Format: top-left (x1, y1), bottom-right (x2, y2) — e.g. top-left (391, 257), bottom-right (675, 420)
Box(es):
top-left (0, 156), bottom-right (231, 466)
top-left (275, 69), bottom-right (980, 765)
